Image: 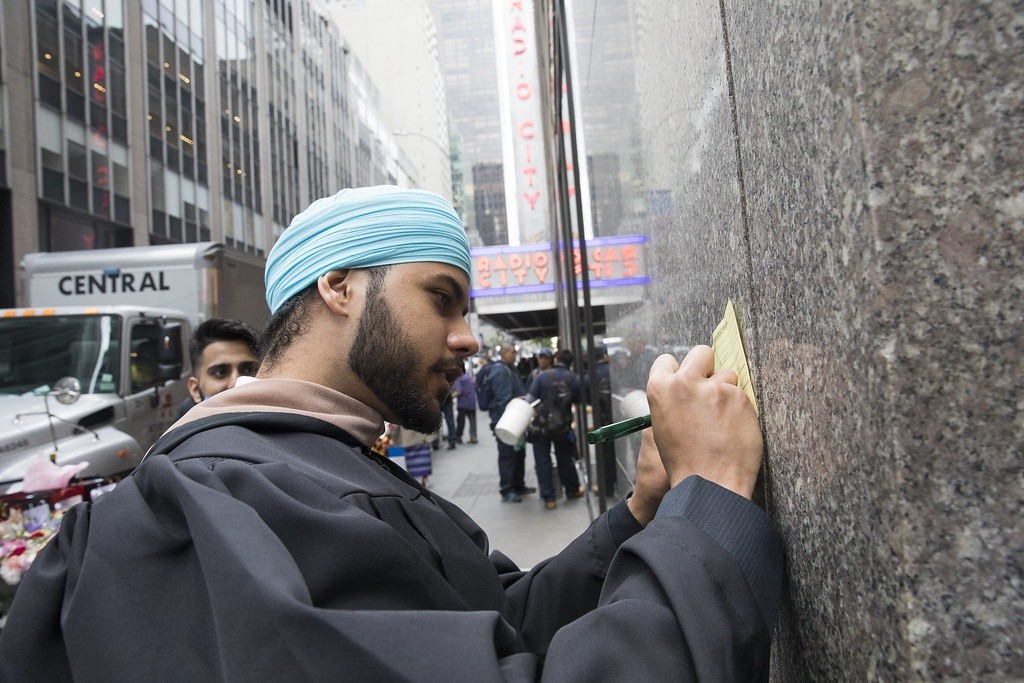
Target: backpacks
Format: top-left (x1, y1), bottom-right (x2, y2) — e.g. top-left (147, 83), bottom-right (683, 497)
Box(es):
top-left (476, 360), bottom-right (506, 411)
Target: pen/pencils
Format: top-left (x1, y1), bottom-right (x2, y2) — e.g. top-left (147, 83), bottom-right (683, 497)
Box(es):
top-left (585, 414), bottom-right (651, 446)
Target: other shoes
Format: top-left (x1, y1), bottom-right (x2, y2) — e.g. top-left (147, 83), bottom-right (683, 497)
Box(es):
top-left (546, 500), bottom-right (557, 509)
top-left (516, 486), bottom-right (536, 495)
top-left (467, 440), bottom-right (478, 444)
top-left (570, 486), bottom-right (585, 497)
top-left (431, 440), bottom-right (439, 450)
top-left (502, 492), bottom-right (522, 503)
top-left (454, 434), bottom-right (462, 443)
top-left (448, 443), bottom-right (455, 449)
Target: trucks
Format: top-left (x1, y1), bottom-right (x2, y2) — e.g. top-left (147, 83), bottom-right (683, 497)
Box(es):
top-left (0, 237), bottom-right (270, 528)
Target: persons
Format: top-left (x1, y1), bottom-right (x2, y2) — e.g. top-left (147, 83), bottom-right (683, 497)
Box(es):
top-left (585, 343), bottom-right (692, 497)
top-left (0, 186), bottom-right (784, 682)
top-left (168, 317), bottom-right (260, 427)
top-left (370, 332), bottom-right (585, 509)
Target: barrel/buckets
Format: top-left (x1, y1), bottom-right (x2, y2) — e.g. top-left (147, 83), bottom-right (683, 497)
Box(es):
top-left (493, 397), bottom-right (542, 445)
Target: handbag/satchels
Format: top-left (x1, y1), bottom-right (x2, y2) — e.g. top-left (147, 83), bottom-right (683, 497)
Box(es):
top-left (523, 403), bottom-right (568, 441)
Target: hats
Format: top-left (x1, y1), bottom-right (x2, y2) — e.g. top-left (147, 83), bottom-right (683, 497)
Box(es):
top-left (265, 185), bottom-right (472, 316)
top-left (539, 348), bottom-right (552, 358)
